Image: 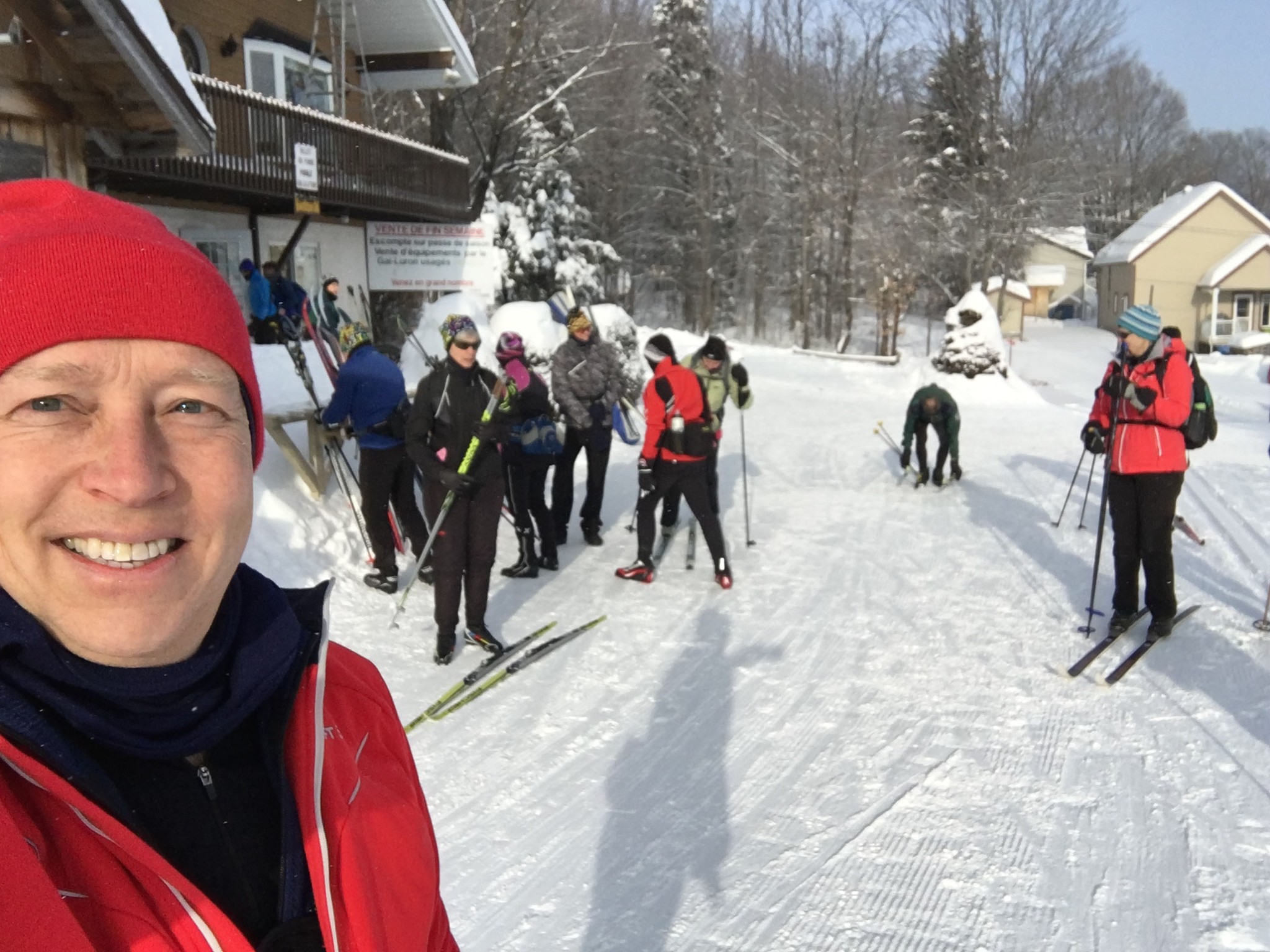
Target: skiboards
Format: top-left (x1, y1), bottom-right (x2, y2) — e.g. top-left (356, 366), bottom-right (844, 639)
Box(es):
top-left (1066, 602), bottom-right (1201, 685)
top-left (649, 515), bottom-right (699, 572)
top-left (281, 298), bottom-right (407, 567)
top-left (401, 612), bottom-right (612, 736)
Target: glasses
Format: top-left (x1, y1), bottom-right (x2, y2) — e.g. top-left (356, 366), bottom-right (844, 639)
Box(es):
top-left (1118, 331), bottom-right (1133, 339)
top-left (450, 337), bottom-right (480, 351)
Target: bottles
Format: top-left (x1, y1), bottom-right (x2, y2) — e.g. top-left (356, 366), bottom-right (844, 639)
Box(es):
top-left (670, 410), bottom-right (685, 435)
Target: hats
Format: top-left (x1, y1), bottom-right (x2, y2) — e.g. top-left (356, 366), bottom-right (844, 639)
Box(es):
top-left (702, 334), bottom-right (725, 360)
top-left (565, 309), bottom-right (592, 334)
top-left (438, 313), bottom-right (475, 349)
top-left (0, 178), bottom-right (264, 473)
top-left (238, 258), bottom-right (254, 273)
top-left (324, 272), bottom-right (340, 286)
top-left (1118, 304), bottom-right (1160, 341)
top-left (338, 321), bottom-right (369, 360)
top-left (644, 334), bottom-right (673, 364)
top-left (496, 332), bottom-right (524, 361)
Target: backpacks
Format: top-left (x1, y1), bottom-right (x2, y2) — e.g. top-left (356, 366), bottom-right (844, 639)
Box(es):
top-left (1113, 351), bottom-right (1217, 448)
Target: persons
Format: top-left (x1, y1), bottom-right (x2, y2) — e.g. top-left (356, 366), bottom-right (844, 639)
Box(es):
top-left (615, 333), bottom-right (733, 589)
top-left (1080, 303), bottom-right (1195, 637)
top-left (551, 305), bottom-right (626, 546)
top-left (238, 258), bottom-right (277, 345)
top-left (305, 276), bottom-right (341, 341)
top-left (660, 335), bottom-right (754, 537)
top-left (402, 313), bottom-right (515, 665)
top-left (899, 383), bottom-right (962, 487)
top-left (495, 331), bottom-right (557, 578)
top-left (312, 321), bottom-right (434, 594)
top-left (0, 177), bottom-right (460, 952)
top-left (262, 260), bottom-right (302, 344)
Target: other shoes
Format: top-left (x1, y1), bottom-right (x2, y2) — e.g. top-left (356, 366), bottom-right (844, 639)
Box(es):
top-left (916, 467), bottom-right (943, 486)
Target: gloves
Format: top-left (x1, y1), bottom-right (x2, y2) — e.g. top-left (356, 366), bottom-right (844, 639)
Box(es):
top-left (1103, 375), bottom-right (1157, 414)
top-left (950, 461), bottom-right (962, 480)
top-left (439, 421), bottom-right (499, 493)
top-left (901, 448), bottom-right (910, 467)
top-left (1081, 422), bottom-right (1106, 454)
top-left (589, 403), bottom-right (604, 420)
top-left (638, 456), bottom-right (657, 491)
top-left (730, 363), bottom-right (748, 388)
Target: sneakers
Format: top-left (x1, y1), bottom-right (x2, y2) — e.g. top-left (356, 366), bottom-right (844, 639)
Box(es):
top-left (1108, 601), bottom-right (1172, 640)
top-left (364, 560), bottom-right (434, 593)
top-left (463, 626), bottom-right (502, 653)
top-left (435, 630), bottom-right (456, 664)
top-left (500, 515), bottom-right (731, 588)
